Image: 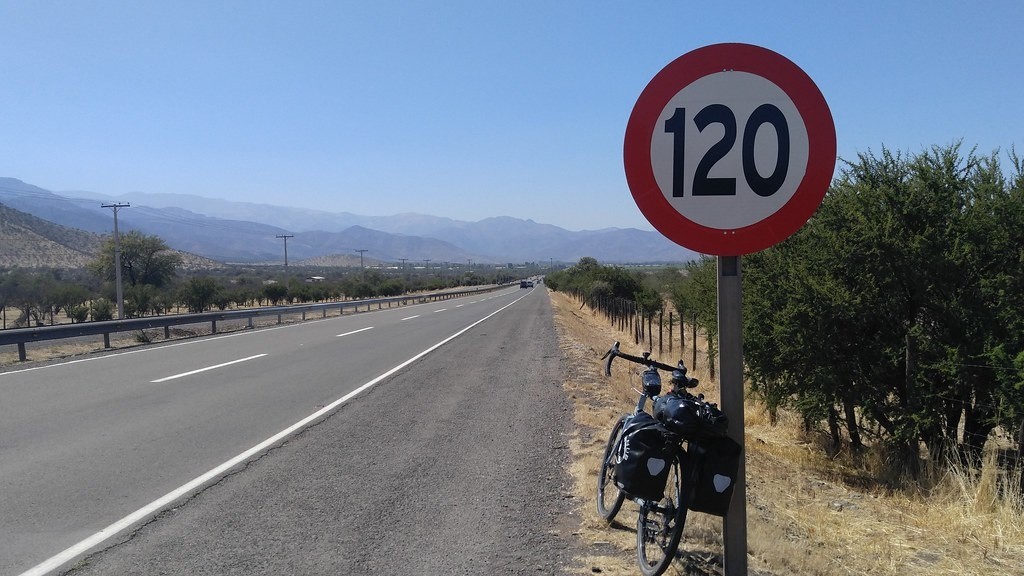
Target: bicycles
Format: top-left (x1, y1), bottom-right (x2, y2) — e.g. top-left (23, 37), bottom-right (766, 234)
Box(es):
top-left (595, 339), bottom-right (729, 576)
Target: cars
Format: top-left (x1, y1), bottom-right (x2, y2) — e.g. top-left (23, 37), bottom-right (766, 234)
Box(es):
top-left (520, 280), bottom-right (535, 289)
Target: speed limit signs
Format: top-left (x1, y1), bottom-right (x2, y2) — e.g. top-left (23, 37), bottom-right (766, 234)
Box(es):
top-left (621, 40), bottom-right (839, 255)
